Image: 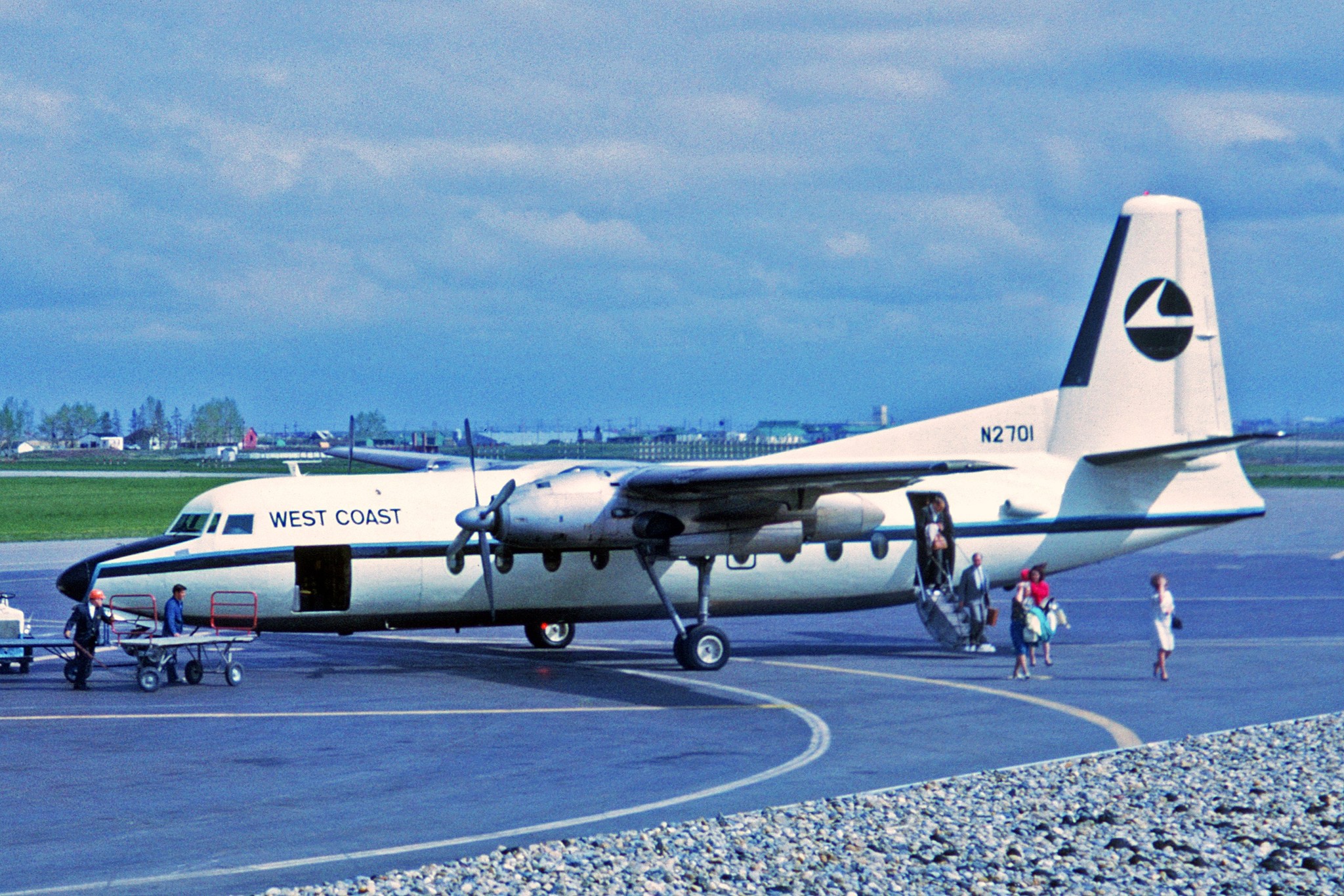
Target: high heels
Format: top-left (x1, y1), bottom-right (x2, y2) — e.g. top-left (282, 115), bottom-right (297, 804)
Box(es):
top-left (1153, 661), bottom-right (1169, 681)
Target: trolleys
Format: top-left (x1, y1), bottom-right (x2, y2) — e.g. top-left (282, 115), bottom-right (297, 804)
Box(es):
top-left (0, 590), bottom-right (110, 682)
top-left (112, 589), bottom-right (259, 691)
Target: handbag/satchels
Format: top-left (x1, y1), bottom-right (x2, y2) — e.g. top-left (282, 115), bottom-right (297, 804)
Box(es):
top-left (1172, 612), bottom-right (1182, 630)
top-left (985, 607), bottom-right (999, 627)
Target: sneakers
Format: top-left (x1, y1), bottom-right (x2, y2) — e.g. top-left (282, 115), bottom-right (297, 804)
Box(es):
top-left (965, 644), bottom-right (996, 652)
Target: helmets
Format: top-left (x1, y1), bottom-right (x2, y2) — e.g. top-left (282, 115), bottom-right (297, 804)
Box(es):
top-left (89, 589), bottom-right (108, 601)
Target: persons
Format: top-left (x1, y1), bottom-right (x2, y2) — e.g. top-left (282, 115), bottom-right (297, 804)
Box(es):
top-left (917, 493), bottom-right (947, 596)
top-left (162, 584), bottom-right (190, 687)
top-left (959, 553), bottom-right (994, 650)
top-left (1149, 573), bottom-right (1178, 679)
top-left (1011, 581), bottom-right (1035, 681)
top-left (64, 589), bottom-right (116, 692)
top-left (1021, 563), bottom-right (1057, 670)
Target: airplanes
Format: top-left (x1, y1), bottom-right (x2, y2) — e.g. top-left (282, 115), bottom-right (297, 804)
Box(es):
top-left (56, 188), bottom-right (1295, 671)
